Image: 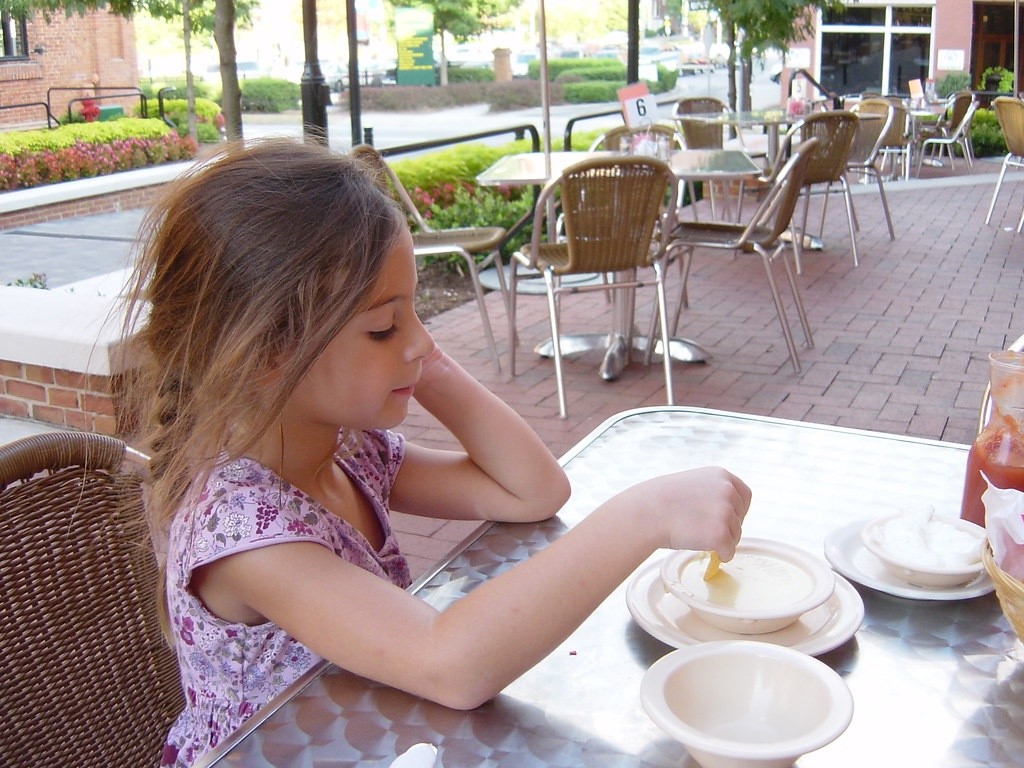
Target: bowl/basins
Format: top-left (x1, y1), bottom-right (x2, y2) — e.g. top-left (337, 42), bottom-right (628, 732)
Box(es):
top-left (641, 640), bottom-right (854, 767)
top-left (860, 515), bottom-right (988, 590)
top-left (661, 536), bottom-right (835, 635)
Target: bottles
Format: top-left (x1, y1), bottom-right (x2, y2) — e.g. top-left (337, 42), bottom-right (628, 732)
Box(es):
top-left (619, 132), bottom-right (669, 167)
top-left (960, 351), bottom-right (1024, 529)
top-left (786, 96), bottom-right (812, 121)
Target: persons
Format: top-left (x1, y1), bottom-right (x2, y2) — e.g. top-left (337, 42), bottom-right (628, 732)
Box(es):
top-left (67, 136), bottom-right (753, 768)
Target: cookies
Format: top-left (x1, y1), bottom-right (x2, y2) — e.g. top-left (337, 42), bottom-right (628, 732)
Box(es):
top-left (696, 551), bottom-right (722, 580)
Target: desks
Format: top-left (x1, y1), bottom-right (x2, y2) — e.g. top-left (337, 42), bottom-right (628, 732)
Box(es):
top-left (477, 150), bottom-right (763, 382)
top-left (201, 403), bottom-right (1023, 767)
top-left (671, 112), bottom-right (883, 249)
top-left (891, 108), bottom-right (943, 181)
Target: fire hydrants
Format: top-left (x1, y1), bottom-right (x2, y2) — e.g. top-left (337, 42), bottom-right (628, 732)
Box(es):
top-left (80, 99), bottom-right (101, 122)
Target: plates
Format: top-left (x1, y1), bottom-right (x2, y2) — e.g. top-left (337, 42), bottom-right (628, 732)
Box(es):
top-left (825, 514), bottom-right (995, 600)
top-left (626, 548), bottom-right (864, 657)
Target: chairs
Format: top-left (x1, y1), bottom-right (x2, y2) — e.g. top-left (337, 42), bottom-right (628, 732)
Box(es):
top-left (0, 432), bottom-right (194, 768)
top-left (343, 76), bottom-right (1024, 419)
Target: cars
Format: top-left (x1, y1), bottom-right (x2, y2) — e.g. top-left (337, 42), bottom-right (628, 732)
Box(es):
top-left (317, 42), bottom-right (741, 93)
